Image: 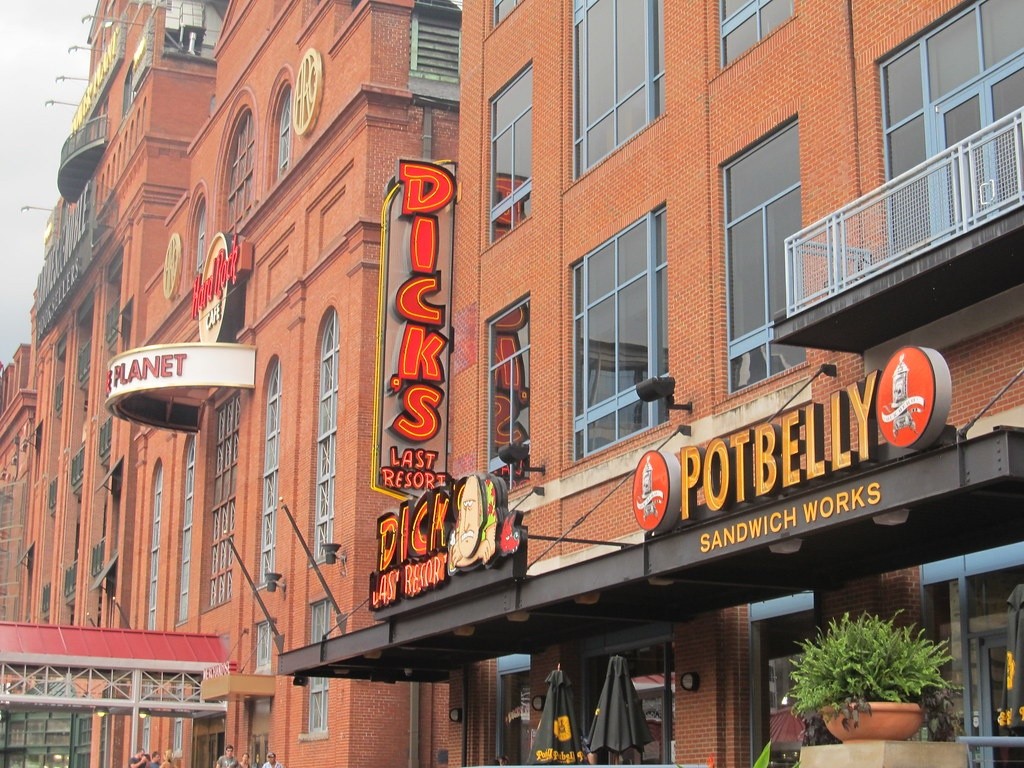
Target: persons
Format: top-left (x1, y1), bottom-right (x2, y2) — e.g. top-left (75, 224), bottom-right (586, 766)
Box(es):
top-left (128, 748), bottom-right (161, 768)
top-left (215, 745), bottom-right (283, 768)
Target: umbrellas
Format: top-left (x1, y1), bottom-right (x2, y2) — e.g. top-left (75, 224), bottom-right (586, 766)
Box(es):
top-left (526, 662), bottom-right (591, 764)
top-left (996, 584), bottom-right (1024, 741)
top-left (587, 655), bottom-right (655, 765)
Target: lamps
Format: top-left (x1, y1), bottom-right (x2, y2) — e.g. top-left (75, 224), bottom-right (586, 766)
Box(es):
top-left (636, 377), bottom-right (692, 410)
top-left (139, 708), bottom-right (151, 719)
top-left (449, 708), bottom-right (462, 723)
top-left (322, 543), bottom-right (347, 565)
top-left (532, 695), bottom-right (546, 711)
top-left (498, 442), bottom-right (546, 475)
top-left (264, 573), bottom-right (286, 592)
top-left (94, 706), bottom-right (110, 717)
top-left (680, 671), bottom-right (700, 692)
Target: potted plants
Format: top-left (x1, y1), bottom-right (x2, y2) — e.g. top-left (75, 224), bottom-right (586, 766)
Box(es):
top-left (784, 608), bottom-right (967, 743)
